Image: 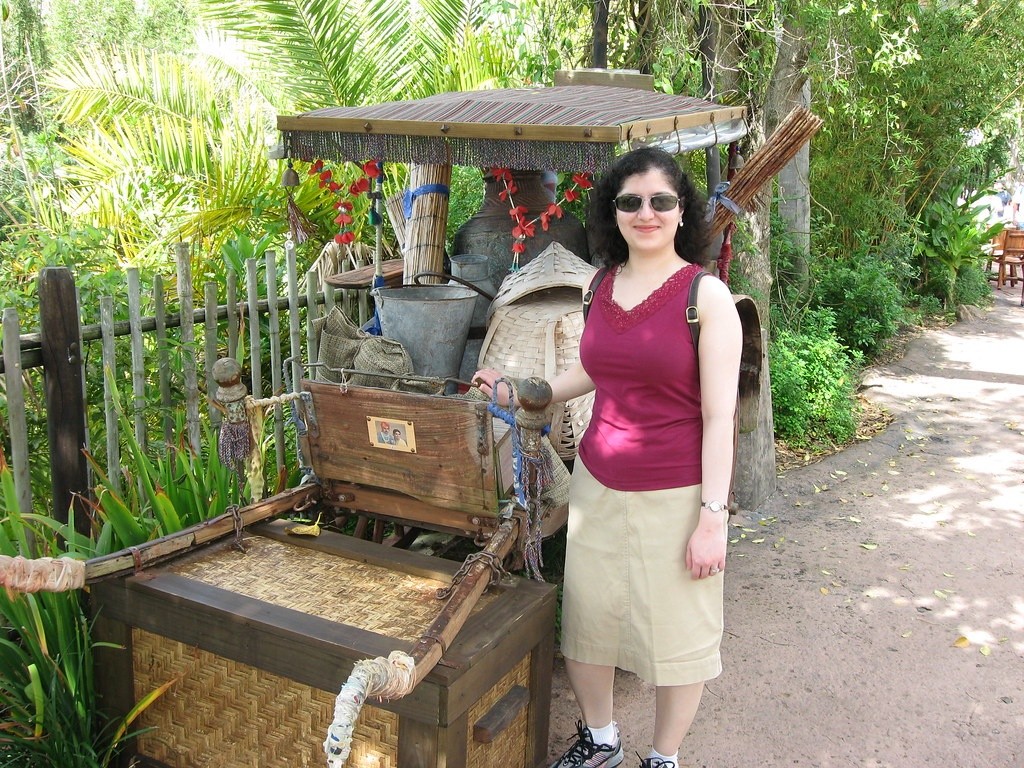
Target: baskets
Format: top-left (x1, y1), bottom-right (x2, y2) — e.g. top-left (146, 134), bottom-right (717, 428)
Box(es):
top-left (478, 288), bottom-right (595, 461)
top-left (485, 241), bottom-right (599, 327)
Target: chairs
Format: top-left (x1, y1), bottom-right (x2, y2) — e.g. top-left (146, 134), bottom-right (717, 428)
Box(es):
top-left (986, 227), bottom-right (1024, 307)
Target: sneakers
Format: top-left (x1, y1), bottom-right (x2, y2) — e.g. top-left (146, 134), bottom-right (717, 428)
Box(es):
top-left (548, 718), bottom-right (625, 768)
top-left (633, 751), bottom-right (680, 768)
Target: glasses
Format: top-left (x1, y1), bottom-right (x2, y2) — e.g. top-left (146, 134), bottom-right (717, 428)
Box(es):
top-left (613, 193), bottom-right (681, 213)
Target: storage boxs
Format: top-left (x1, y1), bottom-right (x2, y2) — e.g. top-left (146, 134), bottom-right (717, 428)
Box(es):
top-left (80, 516), bottom-right (561, 768)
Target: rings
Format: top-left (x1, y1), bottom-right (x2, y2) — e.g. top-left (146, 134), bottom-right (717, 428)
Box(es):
top-left (712, 569), bottom-right (719, 573)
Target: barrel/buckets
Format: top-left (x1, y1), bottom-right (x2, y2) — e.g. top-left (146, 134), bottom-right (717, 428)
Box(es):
top-left (370, 284), bottom-right (479, 397)
top-left (447, 253), bottom-right (498, 385)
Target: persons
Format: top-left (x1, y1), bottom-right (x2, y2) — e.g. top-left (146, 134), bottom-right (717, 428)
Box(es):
top-left (959, 180), bottom-right (1024, 233)
top-left (392, 429), bottom-right (406, 447)
top-left (377, 422), bottom-right (394, 444)
top-left (471, 147), bottom-right (743, 768)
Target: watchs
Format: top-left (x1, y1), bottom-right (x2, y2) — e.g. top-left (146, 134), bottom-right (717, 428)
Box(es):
top-left (701, 501), bottom-right (729, 512)
